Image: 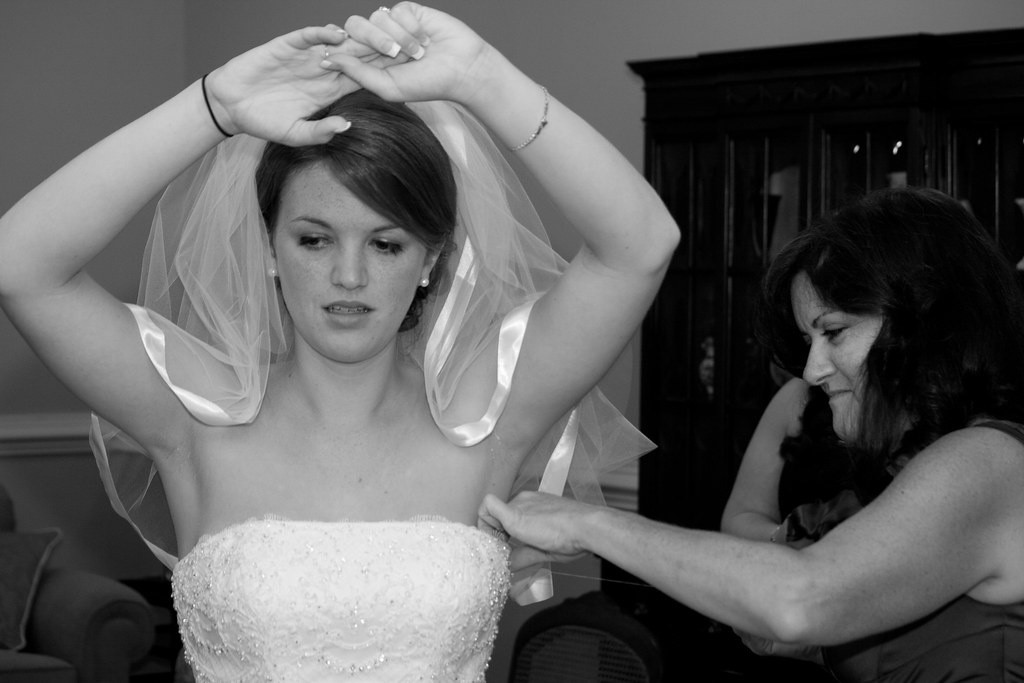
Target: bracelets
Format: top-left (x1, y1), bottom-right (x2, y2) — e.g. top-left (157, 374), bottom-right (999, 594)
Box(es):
top-left (202, 73), bottom-right (234, 138)
top-left (512, 83), bottom-right (549, 150)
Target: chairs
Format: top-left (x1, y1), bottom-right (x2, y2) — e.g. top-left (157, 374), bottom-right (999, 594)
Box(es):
top-left (506, 591), bottom-right (664, 683)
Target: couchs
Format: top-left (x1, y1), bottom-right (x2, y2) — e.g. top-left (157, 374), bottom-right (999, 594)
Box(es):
top-left (0, 484), bottom-right (154, 683)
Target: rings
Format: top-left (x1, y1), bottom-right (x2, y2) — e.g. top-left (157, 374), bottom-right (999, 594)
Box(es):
top-left (324, 47), bottom-right (330, 59)
top-left (380, 6), bottom-right (392, 13)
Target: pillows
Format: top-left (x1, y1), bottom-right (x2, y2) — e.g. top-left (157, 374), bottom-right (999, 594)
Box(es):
top-left (0, 526), bottom-right (63, 652)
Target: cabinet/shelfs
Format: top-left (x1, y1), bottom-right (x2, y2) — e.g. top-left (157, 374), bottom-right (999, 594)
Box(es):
top-left (593, 28), bottom-right (1024, 683)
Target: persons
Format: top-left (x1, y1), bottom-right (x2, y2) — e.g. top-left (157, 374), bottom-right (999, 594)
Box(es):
top-left (479, 188), bottom-right (1024, 683)
top-left (1, 0), bottom-right (682, 683)
